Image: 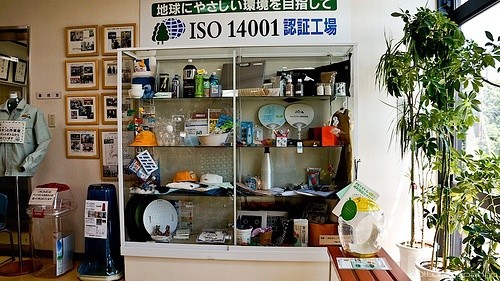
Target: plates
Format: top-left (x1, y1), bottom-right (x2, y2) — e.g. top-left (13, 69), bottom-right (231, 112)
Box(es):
top-left (143, 199), bottom-right (178, 236)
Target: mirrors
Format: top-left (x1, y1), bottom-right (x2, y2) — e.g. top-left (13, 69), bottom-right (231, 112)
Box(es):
top-left (117, 43), bottom-right (359, 261)
top-left (0, 25), bottom-right (33, 256)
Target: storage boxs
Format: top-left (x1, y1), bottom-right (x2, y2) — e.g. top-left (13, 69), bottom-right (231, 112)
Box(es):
top-left (323, 197), bottom-right (338, 223)
top-left (293, 218), bottom-right (308, 247)
top-left (309, 221), bottom-right (338, 247)
top-left (313, 126), bottom-right (338, 148)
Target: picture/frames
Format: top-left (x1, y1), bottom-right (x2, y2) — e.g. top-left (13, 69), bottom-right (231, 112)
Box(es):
top-left (98, 129), bottom-right (138, 180)
top-left (65, 95), bottom-right (99, 125)
top-left (65, 59), bottom-right (99, 90)
top-left (65, 25), bottom-right (99, 56)
top-left (0, 59), bottom-right (10, 81)
top-left (102, 58), bottom-right (135, 90)
top-left (101, 92), bottom-right (138, 125)
top-left (13, 59), bottom-right (26, 83)
top-left (102, 22), bottom-right (136, 55)
top-left (65, 127), bottom-right (100, 159)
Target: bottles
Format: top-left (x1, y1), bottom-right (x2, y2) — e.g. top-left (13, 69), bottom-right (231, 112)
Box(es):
top-left (316, 83), bottom-right (332, 96)
top-left (159, 73), bottom-right (170, 92)
top-left (260, 147), bottom-right (274, 190)
top-left (246, 124), bottom-right (252, 145)
top-left (183, 58), bottom-right (219, 97)
top-left (171, 75), bottom-right (180, 98)
top-left (281, 67), bottom-right (303, 97)
top-left (253, 124), bottom-right (263, 144)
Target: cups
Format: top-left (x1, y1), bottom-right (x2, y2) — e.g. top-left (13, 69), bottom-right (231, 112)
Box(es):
top-left (131, 83), bottom-right (142, 91)
top-left (235, 226), bottom-right (253, 244)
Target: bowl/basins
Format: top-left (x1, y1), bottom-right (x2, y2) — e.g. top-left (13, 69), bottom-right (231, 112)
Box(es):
top-left (127, 90), bottom-right (145, 98)
top-left (198, 132), bottom-right (230, 146)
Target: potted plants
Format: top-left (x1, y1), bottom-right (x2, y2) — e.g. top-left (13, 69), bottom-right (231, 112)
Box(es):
top-left (374, 0), bottom-right (500, 281)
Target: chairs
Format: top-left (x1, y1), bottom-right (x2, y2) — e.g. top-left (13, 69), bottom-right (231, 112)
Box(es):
top-left (0, 193), bottom-right (15, 269)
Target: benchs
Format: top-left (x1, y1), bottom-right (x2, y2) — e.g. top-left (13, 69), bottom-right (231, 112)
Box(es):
top-left (327, 245), bottom-right (412, 281)
